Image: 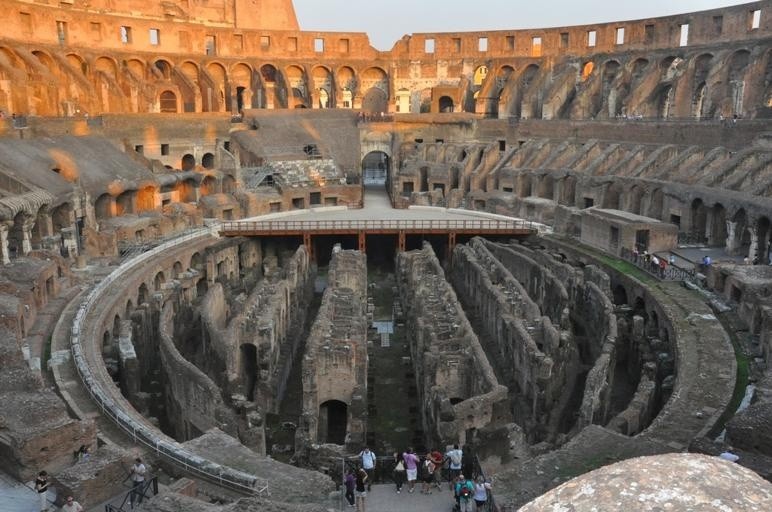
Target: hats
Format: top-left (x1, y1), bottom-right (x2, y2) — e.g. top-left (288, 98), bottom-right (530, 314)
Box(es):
top-left (424, 453), bottom-right (433, 460)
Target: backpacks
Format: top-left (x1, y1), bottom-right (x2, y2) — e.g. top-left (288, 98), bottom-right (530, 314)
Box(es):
top-left (422, 462), bottom-right (432, 479)
top-left (459, 481), bottom-right (471, 498)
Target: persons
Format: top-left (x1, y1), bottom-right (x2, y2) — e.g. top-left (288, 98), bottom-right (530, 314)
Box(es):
top-left (126, 458), bottom-right (146, 506)
top-left (703, 256), bottom-right (711, 274)
top-left (632, 247), bottom-right (675, 278)
top-left (59, 496), bottom-right (84, 512)
top-left (35, 470), bottom-right (52, 512)
top-left (357, 111), bottom-right (385, 123)
top-left (732, 113), bottom-right (737, 123)
top-left (78, 445), bottom-right (92, 462)
top-left (752, 253), bottom-right (761, 265)
top-left (342, 444), bottom-right (492, 512)
top-left (720, 114), bottom-right (724, 122)
top-left (743, 255), bottom-right (752, 265)
